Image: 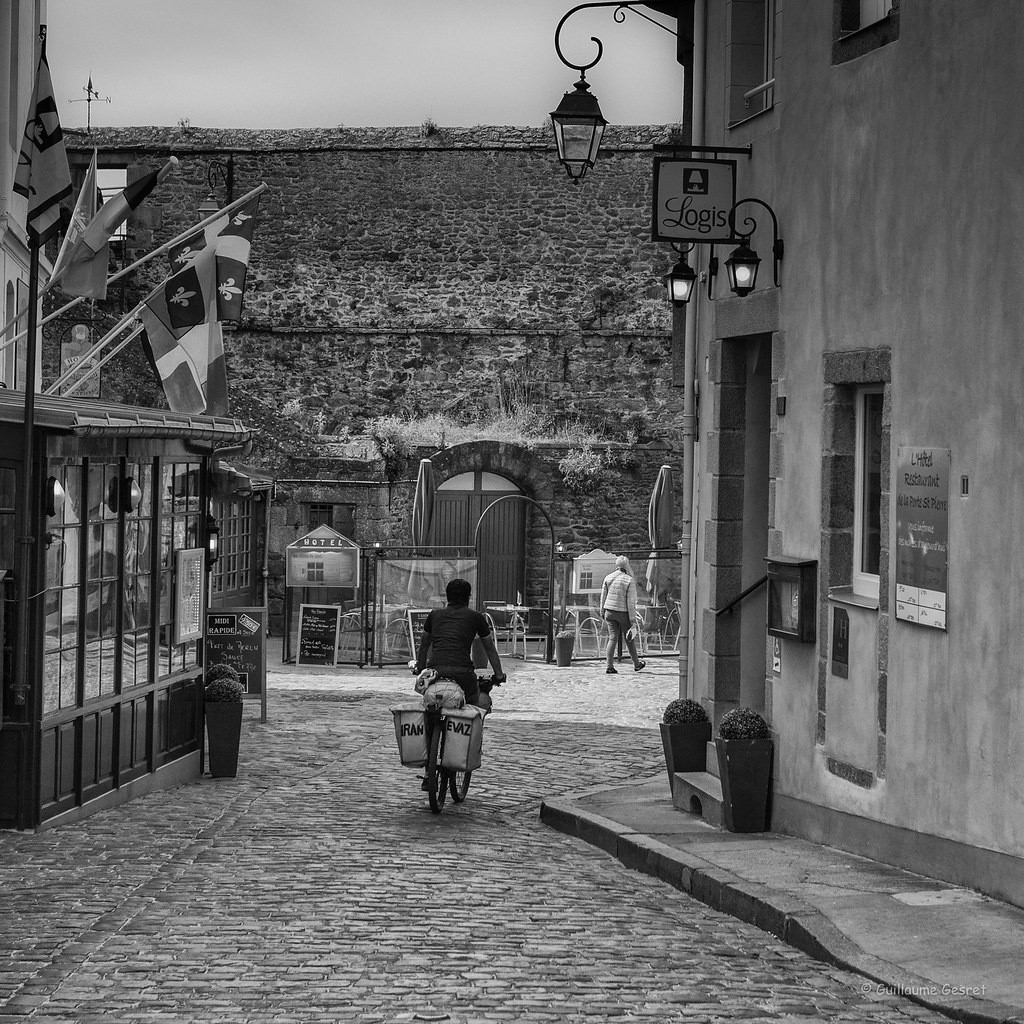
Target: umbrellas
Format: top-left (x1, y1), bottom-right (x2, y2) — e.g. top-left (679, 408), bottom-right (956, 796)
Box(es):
top-left (646, 466), bottom-right (674, 606)
top-left (407, 458), bottom-right (437, 607)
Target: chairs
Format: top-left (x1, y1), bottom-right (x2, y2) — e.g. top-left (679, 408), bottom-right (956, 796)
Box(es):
top-left (333, 601), bottom-right (412, 662)
top-left (480, 598), bottom-right (681, 662)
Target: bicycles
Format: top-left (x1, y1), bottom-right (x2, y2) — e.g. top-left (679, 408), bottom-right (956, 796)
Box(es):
top-left (409, 661), bottom-right (509, 816)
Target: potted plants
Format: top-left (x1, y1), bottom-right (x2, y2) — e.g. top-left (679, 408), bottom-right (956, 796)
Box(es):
top-left (472, 633), bottom-right (488, 668)
top-left (659, 699), bottom-right (712, 796)
top-left (715, 708), bottom-right (774, 833)
top-left (554, 630), bottom-right (575, 667)
top-left (203, 664), bottom-right (245, 778)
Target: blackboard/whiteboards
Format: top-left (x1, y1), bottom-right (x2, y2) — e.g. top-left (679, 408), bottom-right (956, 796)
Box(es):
top-left (295, 604), bottom-right (342, 667)
top-left (202, 606), bottom-right (267, 700)
top-left (399, 609), bottom-right (432, 660)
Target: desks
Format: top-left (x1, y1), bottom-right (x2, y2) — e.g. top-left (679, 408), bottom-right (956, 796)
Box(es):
top-left (484, 607), bottom-right (549, 660)
top-left (553, 606), bottom-right (601, 659)
top-left (348, 605), bottom-right (398, 661)
top-left (634, 604), bottom-right (666, 656)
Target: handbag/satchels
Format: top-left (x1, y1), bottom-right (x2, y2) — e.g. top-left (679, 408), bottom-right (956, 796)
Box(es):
top-left (389, 702), bottom-right (428, 769)
top-left (626, 627), bottom-right (637, 643)
top-left (441, 704), bottom-right (488, 772)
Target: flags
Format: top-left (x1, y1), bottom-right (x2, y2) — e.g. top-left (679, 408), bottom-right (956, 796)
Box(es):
top-left (55, 149), bottom-right (110, 301)
top-left (164, 193), bottom-right (262, 330)
top-left (137, 246), bottom-right (230, 418)
top-left (13, 45), bottom-right (74, 249)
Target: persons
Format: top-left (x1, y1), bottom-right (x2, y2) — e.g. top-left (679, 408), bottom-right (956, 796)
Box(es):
top-left (412, 579), bottom-right (504, 790)
top-left (600, 555), bottom-right (646, 674)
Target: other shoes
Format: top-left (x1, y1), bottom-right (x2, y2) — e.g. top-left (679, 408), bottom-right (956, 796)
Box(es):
top-left (634, 661), bottom-right (646, 671)
top-left (606, 668), bottom-right (618, 674)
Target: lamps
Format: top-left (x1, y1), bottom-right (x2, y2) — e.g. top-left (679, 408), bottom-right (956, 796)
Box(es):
top-left (104, 476), bottom-right (118, 513)
top-left (195, 151), bottom-right (239, 224)
top-left (206, 512), bottom-right (221, 572)
top-left (547, 0), bottom-right (696, 186)
top-left (123, 477), bottom-right (142, 514)
top-left (723, 198), bottom-right (785, 298)
top-left (663, 241), bottom-right (718, 309)
top-left (46, 476), bottom-right (65, 518)
